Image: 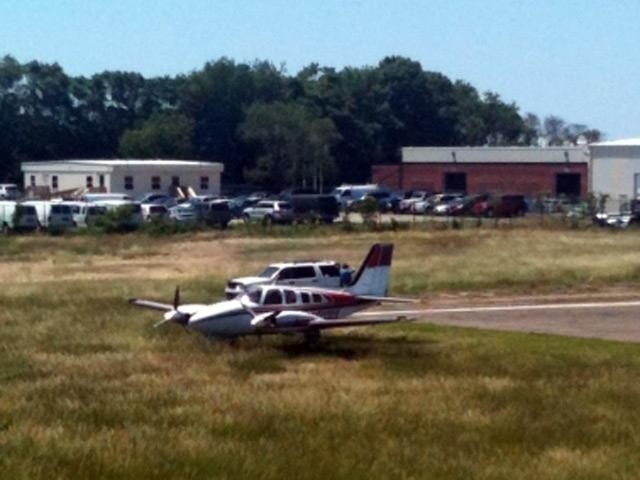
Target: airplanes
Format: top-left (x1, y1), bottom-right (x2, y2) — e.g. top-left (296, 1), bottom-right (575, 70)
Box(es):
top-left (128, 242), bottom-right (424, 345)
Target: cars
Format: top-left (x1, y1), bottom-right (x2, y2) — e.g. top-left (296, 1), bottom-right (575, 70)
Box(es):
top-left (224, 260), bottom-right (341, 299)
top-left (345, 189), bottom-right (527, 218)
top-left (231, 194), bottom-right (339, 225)
top-left (0, 183), bottom-right (21, 198)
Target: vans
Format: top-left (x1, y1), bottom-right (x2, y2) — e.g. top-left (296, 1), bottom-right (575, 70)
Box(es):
top-left (0, 194), bottom-right (231, 235)
top-left (331, 184), bottom-right (377, 211)
